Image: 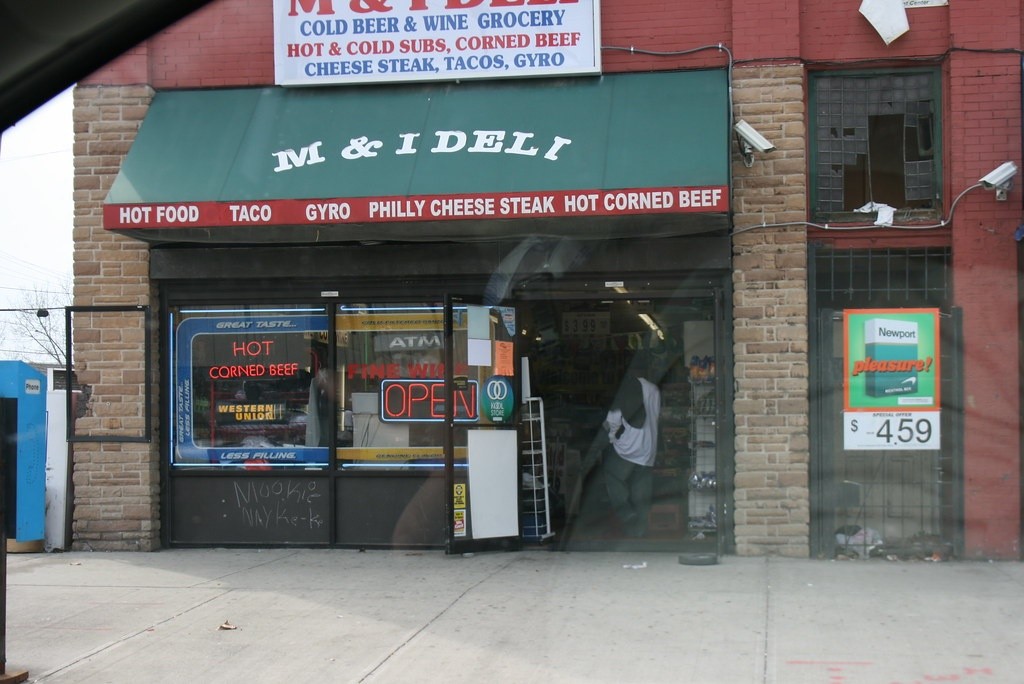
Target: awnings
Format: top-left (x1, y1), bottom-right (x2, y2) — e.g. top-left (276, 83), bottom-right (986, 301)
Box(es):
top-left (102, 63), bottom-right (732, 241)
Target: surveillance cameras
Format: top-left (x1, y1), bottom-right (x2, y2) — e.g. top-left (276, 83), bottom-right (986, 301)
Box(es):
top-left (978, 160), bottom-right (1019, 190)
top-left (733, 119), bottom-right (777, 154)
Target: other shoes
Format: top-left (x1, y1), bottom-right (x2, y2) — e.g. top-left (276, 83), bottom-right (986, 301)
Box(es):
top-left (599, 528), bottom-right (624, 539)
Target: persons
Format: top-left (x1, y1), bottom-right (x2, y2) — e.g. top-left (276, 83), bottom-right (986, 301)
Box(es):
top-left (603, 355), bottom-right (660, 541)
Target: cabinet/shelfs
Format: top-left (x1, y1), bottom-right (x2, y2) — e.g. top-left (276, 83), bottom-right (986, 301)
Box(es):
top-left (688, 380), bottom-right (717, 534)
